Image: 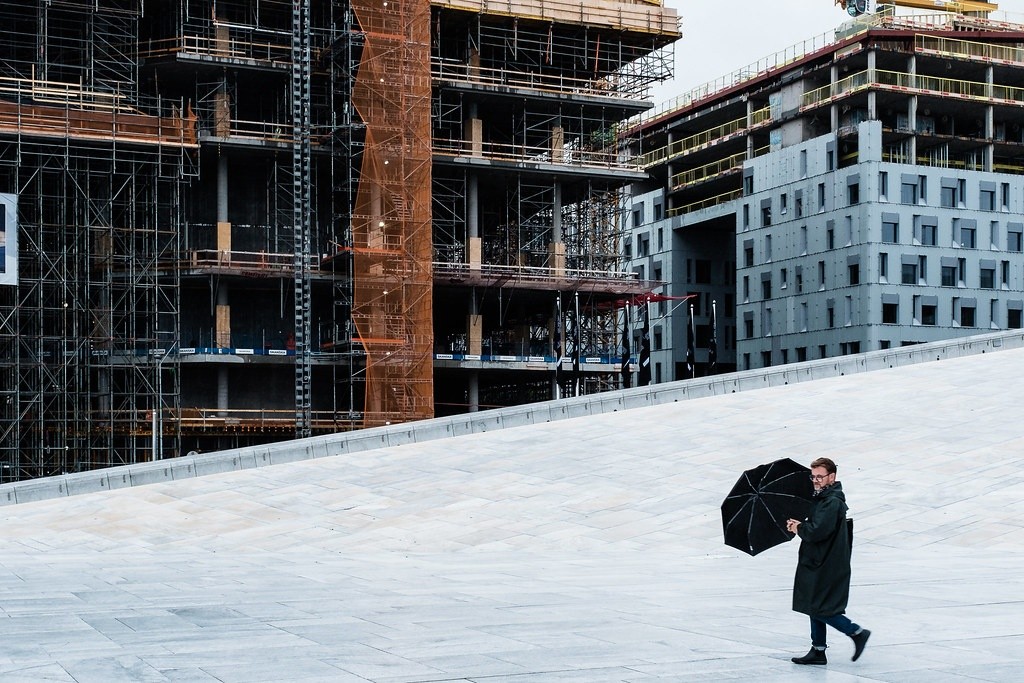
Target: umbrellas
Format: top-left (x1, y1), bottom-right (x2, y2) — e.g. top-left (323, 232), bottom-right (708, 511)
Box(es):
top-left (719, 457), bottom-right (813, 557)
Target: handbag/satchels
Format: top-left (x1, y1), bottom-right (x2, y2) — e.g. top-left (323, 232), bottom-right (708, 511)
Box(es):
top-left (846, 518), bottom-right (853, 557)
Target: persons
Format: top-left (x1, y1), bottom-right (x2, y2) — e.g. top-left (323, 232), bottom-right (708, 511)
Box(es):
top-left (787, 457), bottom-right (871, 665)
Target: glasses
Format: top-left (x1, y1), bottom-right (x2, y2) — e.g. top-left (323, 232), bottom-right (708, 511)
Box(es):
top-left (810, 472), bottom-right (834, 483)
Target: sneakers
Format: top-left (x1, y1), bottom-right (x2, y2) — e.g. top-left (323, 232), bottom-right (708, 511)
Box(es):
top-left (850, 629), bottom-right (870, 661)
top-left (792, 646), bottom-right (827, 665)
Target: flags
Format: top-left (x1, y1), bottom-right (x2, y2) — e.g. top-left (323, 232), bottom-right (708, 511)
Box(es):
top-left (621, 306), bottom-right (630, 387)
top-left (684, 309), bottom-right (696, 371)
top-left (572, 303), bottom-right (579, 382)
top-left (639, 303), bottom-right (651, 386)
top-left (707, 306), bottom-right (718, 367)
top-left (553, 304), bottom-right (564, 389)
